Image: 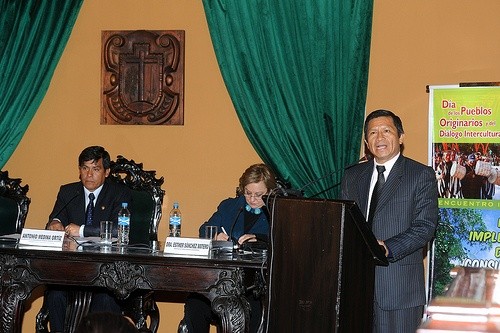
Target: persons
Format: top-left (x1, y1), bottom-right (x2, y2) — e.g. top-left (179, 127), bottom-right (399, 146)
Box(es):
top-left (184, 163), bottom-right (278, 333)
top-left (342, 110), bottom-right (439, 333)
top-left (44, 146), bottom-right (142, 333)
top-left (434, 148), bottom-right (500, 200)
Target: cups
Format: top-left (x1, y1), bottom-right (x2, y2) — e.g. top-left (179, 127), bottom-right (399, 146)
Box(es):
top-left (100, 221), bottom-right (113, 241)
top-left (205, 226), bottom-right (218, 241)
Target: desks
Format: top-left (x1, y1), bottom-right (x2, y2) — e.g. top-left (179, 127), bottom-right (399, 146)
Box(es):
top-left (0, 237), bottom-right (267, 333)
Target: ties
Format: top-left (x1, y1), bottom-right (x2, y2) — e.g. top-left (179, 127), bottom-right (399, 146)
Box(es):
top-left (85, 193), bottom-right (95, 226)
top-left (368, 165), bottom-right (386, 228)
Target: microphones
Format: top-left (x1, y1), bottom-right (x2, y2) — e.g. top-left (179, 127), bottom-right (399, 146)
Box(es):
top-left (45, 190), bottom-right (81, 230)
top-left (276, 153), bottom-right (373, 198)
top-left (212, 207), bottom-right (245, 252)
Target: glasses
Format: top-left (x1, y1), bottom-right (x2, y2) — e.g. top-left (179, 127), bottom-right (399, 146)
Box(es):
top-left (243, 190), bottom-right (268, 199)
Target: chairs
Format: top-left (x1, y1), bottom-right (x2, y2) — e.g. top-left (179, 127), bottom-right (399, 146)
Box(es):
top-left (0, 170), bottom-right (31, 236)
top-left (34, 154), bottom-right (167, 333)
top-left (235, 176), bottom-right (292, 198)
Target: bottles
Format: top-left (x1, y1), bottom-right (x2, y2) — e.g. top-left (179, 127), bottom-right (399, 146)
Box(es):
top-left (168, 203), bottom-right (181, 237)
top-left (117, 202), bottom-right (129, 245)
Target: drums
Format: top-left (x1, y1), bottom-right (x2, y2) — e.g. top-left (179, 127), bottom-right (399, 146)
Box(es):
top-left (475, 160), bottom-right (491, 176)
top-left (488, 168), bottom-right (500, 186)
top-left (450, 163), bottom-right (467, 180)
